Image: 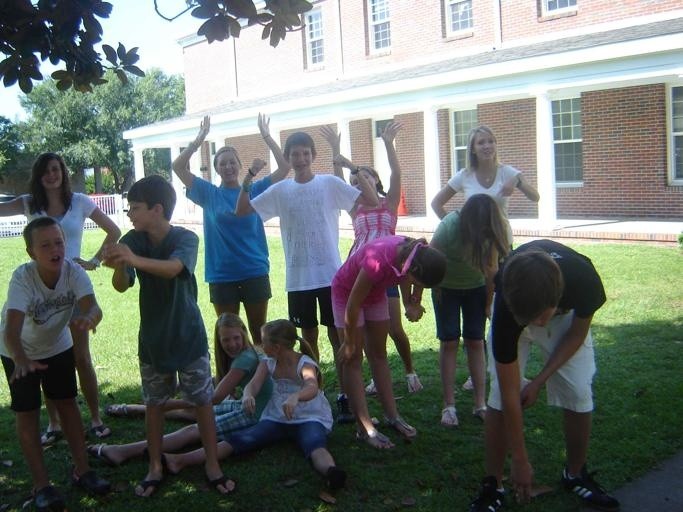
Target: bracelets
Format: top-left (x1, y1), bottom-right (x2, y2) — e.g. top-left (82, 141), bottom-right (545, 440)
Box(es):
top-left (241, 181), bottom-right (254, 193)
top-left (247, 168), bottom-right (258, 178)
top-left (260, 134), bottom-right (274, 140)
top-left (188, 142), bottom-right (201, 151)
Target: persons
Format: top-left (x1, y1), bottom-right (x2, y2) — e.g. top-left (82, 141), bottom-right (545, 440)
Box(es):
top-left (318, 122), bottom-right (424, 395)
top-left (2, 155), bottom-right (118, 447)
top-left (172, 113), bottom-right (292, 346)
top-left (142, 319), bottom-right (348, 494)
top-left (1, 216), bottom-right (113, 510)
top-left (431, 126), bottom-right (540, 230)
top-left (329, 232), bottom-right (448, 451)
top-left (102, 176), bottom-right (236, 501)
top-left (86, 313), bottom-right (275, 465)
top-left (400, 192), bottom-right (497, 427)
top-left (467, 238), bottom-right (620, 512)
top-left (234, 131), bottom-right (378, 425)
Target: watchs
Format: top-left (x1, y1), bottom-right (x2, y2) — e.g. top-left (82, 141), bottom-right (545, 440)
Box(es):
top-left (350, 166), bottom-right (361, 175)
top-left (409, 295), bottom-right (421, 304)
top-left (89, 258), bottom-right (101, 268)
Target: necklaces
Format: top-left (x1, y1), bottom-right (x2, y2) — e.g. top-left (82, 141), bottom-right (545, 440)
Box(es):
top-left (479, 164), bottom-right (496, 186)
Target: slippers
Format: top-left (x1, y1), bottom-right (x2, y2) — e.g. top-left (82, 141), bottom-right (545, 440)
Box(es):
top-left (365, 378), bottom-right (377, 394)
top-left (143, 447), bottom-right (176, 478)
top-left (87, 443), bottom-right (115, 467)
top-left (134, 473), bottom-right (168, 498)
top-left (440, 407), bottom-right (457, 426)
top-left (205, 473), bottom-right (236, 496)
top-left (70, 467), bottom-right (110, 496)
top-left (327, 466), bottom-right (346, 489)
top-left (90, 417), bottom-right (112, 438)
top-left (406, 374), bottom-right (423, 393)
top-left (41, 430), bottom-right (63, 445)
top-left (471, 406), bottom-right (486, 421)
top-left (34, 484), bottom-right (66, 512)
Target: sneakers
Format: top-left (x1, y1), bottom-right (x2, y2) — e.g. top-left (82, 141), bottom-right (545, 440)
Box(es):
top-left (336, 393), bottom-right (356, 422)
top-left (560, 461), bottom-right (621, 512)
top-left (104, 403), bottom-right (129, 416)
top-left (467, 475), bottom-right (505, 512)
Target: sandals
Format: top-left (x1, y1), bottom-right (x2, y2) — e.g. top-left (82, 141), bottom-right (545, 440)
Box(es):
top-left (356, 426), bottom-right (390, 450)
top-left (383, 416), bottom-right (416, 437)
